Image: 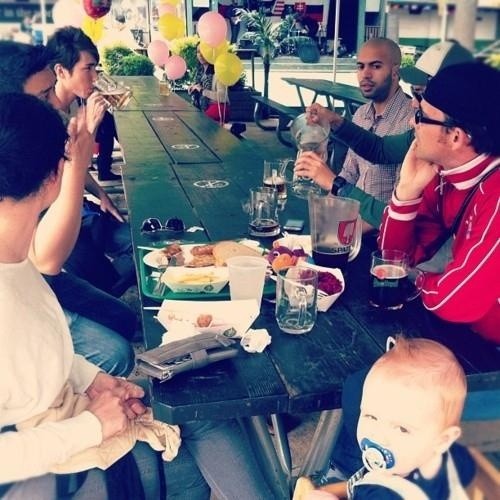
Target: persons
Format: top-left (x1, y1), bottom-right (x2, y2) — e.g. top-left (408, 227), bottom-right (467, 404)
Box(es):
top-left (293, 41), bottom-right (474, 232)
top-left (298, 330), bottom-right (500, 500)
top-left (376, 61), bottom-right (499, 347)
top-left (1, 27), bottom-right (279, 498)
top-left (335, 36), bottom-right (417, 233)
top-left (110, 15), bottom-right (137, 51)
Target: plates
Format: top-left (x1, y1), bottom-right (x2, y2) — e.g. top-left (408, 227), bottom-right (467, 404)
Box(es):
top-left (141, 239), bottom-right (270, 295)
top-left (273, 235), bottom-right (312, 257)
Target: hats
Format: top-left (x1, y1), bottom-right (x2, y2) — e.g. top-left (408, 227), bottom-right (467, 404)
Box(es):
top-left (423, 61), bottom-right (500, 122)
top-left (397, 40), bottom-right (474, 86)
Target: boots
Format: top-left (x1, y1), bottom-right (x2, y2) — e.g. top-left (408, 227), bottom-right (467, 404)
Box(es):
top-left (96, 156), bottom-right (121, 181)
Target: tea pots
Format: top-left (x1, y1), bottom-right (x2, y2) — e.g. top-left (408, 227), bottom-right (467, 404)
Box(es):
top-left (290, 112), bottom-right (331, 164)
top-left (308, 195), bottom-right (363, 268)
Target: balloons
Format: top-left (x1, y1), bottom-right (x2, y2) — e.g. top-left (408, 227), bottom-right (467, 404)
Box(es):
top-left (147, 12), bottom-right (243, 84)
top-left (78, 0), bottom-right (112, 47)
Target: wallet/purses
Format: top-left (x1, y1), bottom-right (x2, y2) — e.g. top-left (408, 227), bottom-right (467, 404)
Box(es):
top-left (135, 330), bottom-right (238, 384)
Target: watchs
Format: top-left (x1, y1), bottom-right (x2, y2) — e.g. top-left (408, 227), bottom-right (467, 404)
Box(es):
top-left (331, 175), bottom-right (347, 197)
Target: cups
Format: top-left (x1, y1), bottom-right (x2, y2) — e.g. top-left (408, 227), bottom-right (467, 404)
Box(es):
top-left (159, 78), bottom-right (169, 96)
top-left (226, 256), bottom-right (266, 305)
top-left (370, 250), bottom-right (425, 310)
top-left (91, 71), bottom-right (133, 112)
top-left (247, 186), bottom-right (280, 239)
top-left (263, 160), bottom-right (289, 211)
top-left (276, 268), bottom-right (320, 334)
top-left (293, 147), bottom-right (330, 199)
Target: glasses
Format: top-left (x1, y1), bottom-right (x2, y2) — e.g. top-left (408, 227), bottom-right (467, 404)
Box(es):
top-left (414, 109), bottom-right (472, 138)
top-left (139, 216), bottom-right (185, 235)
